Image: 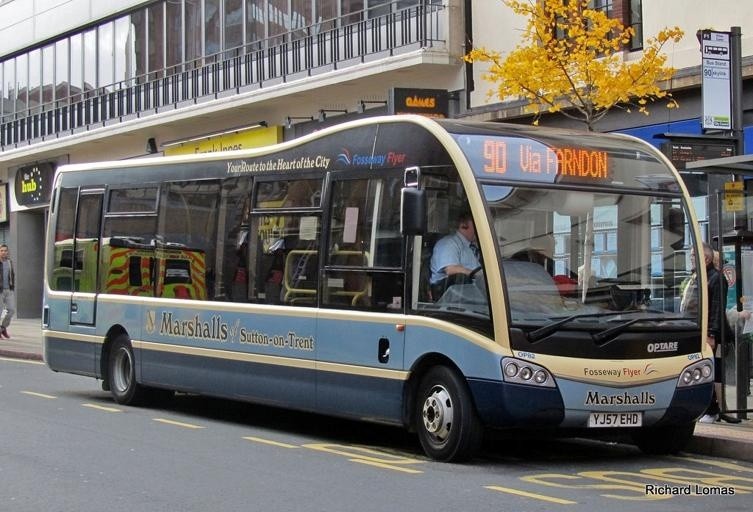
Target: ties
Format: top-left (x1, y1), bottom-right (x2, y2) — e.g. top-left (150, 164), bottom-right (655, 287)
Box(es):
top-left (469, 243), bottom-right (483, 266)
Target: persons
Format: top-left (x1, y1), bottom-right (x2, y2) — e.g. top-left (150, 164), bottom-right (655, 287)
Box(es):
top-left (673, 241), bottom-right (734, 423)
top-left (708, 250), bottom-right (749, 424)
top-left (0, 245), bottom-right (15, 339)
top-left (425, 202), bottom-right (481, 303)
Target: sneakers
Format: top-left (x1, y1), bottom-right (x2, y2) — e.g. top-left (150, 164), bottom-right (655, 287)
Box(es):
top-left (0, 326), bottom-right (10, 340)
top-left (698, 410), bottom-right (742, 424)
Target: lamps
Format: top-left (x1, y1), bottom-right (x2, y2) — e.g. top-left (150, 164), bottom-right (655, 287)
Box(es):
top-left (318, 109), bottom-right (347, 123)
top-left (356, 99), bottom-right (387, 114)
top-left (285, 116), bottom-right (314, 129)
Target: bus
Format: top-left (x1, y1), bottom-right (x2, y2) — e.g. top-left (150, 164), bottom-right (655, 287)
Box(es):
top-left (40, 114), bottom-right (716, 464)
